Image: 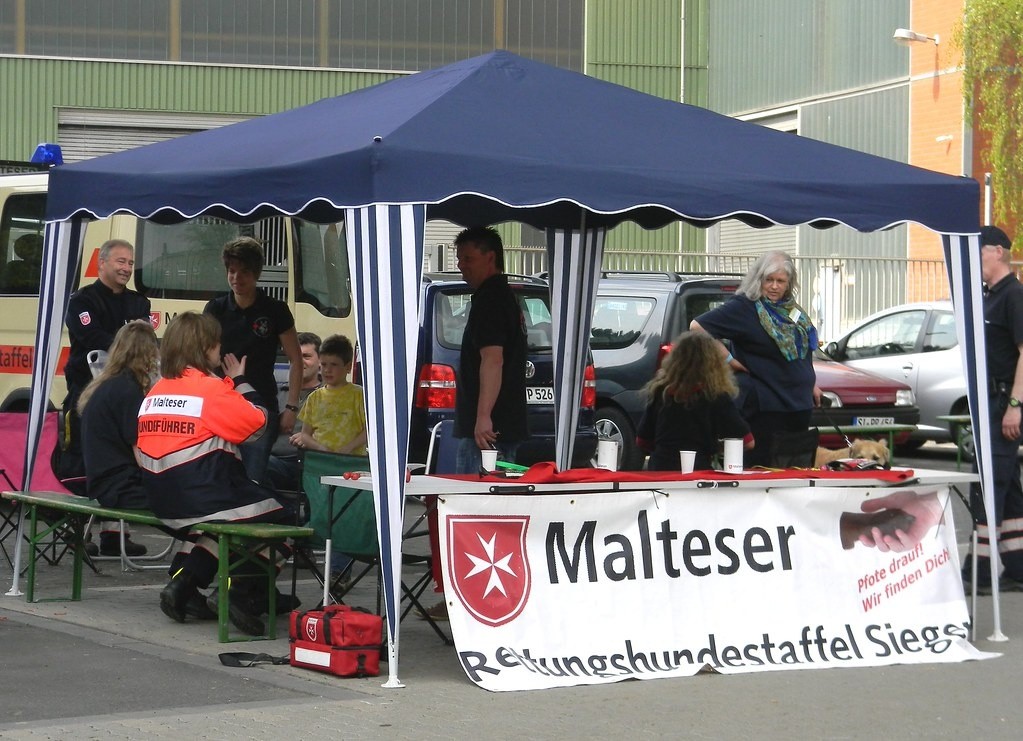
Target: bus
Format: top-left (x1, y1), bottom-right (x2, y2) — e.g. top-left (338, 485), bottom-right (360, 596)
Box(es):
top-left (0, 143), bottom-right (361, 413)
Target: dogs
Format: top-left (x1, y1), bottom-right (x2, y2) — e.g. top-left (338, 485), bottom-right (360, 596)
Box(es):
top-left (814, 438), bottom-right (890, 470)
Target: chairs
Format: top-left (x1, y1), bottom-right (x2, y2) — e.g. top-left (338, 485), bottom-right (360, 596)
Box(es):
top-left (290, 420), bottom-right (465, 658)
top-left (0, 410), bottom-right (103, 578)
top-left (6, 234), bottom-right (42, 293)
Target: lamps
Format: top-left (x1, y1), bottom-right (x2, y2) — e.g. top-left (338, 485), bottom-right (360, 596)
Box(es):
top-left (896, 26), bottom-right (941, 46)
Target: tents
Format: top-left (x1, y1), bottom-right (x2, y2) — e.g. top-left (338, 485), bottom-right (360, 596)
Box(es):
top-left (7, 48), bottom-right (1008, 691)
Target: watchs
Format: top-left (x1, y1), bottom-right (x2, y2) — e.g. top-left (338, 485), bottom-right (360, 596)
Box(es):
top-left (1008, 397), bottom-right (1022, 407)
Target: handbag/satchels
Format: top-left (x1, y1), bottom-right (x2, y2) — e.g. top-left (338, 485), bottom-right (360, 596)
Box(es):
top-left (218, 606), bottom-right (383, 677)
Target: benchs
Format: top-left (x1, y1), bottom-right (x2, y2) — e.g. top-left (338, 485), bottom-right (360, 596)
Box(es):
top-left (2, 486), bottom-right (316, 642)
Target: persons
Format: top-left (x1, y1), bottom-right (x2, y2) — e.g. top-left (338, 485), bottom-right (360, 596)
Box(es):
top-left (962, 227), bottom-right (1023, 596)
top-left (635, 332), bottom-right (755, 471)
top-left (271, 332), bottom-right (324, 568)
top-left (298, 335), bottom-right (368, 591)
top-left (691, 250), bottom-right (822, 469)
top-left (414, 227), bottom-right (528, 620)
top-left (63, 240), bottom-right (150, 556)
top-left (78, 322), bottom-right (220, 621)
top-left (137, 313), bottom-right (306, 634)
top-left (202, 236), bottom-right (303, 484)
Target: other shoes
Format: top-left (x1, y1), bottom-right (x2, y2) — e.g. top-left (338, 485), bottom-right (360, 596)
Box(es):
top-left (963, 579), bottom-right (992, 594)
top-left (66, 533), bottom-right (99, 555)
top-left (414, 600), bottom-right (449, 622)
top-left (100, 535), bottom-right (147, 555)
top-left (320, 568), bottom-right (353, 590)
top-left (297, 549), bottom-right (317, 568)
top-left (999, 576), bottom-right (1023, 592)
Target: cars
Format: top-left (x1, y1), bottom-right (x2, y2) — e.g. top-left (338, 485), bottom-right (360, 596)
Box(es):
top-left (823, 299), bottom-right (974, 459)
top-left (812, 345), bottom-right (919, 451)
top-left (409, 270), bottom-right (598, 466)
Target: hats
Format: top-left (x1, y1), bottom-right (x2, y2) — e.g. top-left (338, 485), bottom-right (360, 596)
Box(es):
top-left (982, 225), bottom-right (1011, 249)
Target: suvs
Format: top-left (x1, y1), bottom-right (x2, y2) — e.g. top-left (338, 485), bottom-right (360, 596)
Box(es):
top-left (525, 266), bottom-right (747, 465)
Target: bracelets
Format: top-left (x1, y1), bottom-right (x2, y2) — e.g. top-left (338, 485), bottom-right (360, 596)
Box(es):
top-left (724, 354), bottom-right (734, 363)
top-left (285, 403), bottom-right (298, 412)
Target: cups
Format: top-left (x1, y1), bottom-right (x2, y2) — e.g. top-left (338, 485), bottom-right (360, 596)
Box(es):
top-left (724, 438), bottom-right (744, 473)
top-left (597, 441), bottom-right (618, 472)
top-left (680, 451), bottom-right (696, 474)
top-left (481, 450), bottom-right (498, 472)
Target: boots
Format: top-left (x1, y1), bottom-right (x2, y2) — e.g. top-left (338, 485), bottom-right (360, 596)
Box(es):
top-left (168, 551), bottom-right (215, 618)
top-left (159, 546), bottom-right (218, 622)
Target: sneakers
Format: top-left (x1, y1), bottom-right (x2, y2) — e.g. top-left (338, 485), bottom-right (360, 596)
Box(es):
top-left (208, 581), bottom-right (266, 636)
top-left (276, 589), bottom-right (300, 614)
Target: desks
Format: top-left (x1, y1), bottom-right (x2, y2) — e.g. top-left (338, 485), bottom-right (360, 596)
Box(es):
top-left (814, 424), bottom-right (917, 463)
top-left (937, 415), bottom-right (971, 468)
top-left (313, 468), bottom-right (982, 657)
